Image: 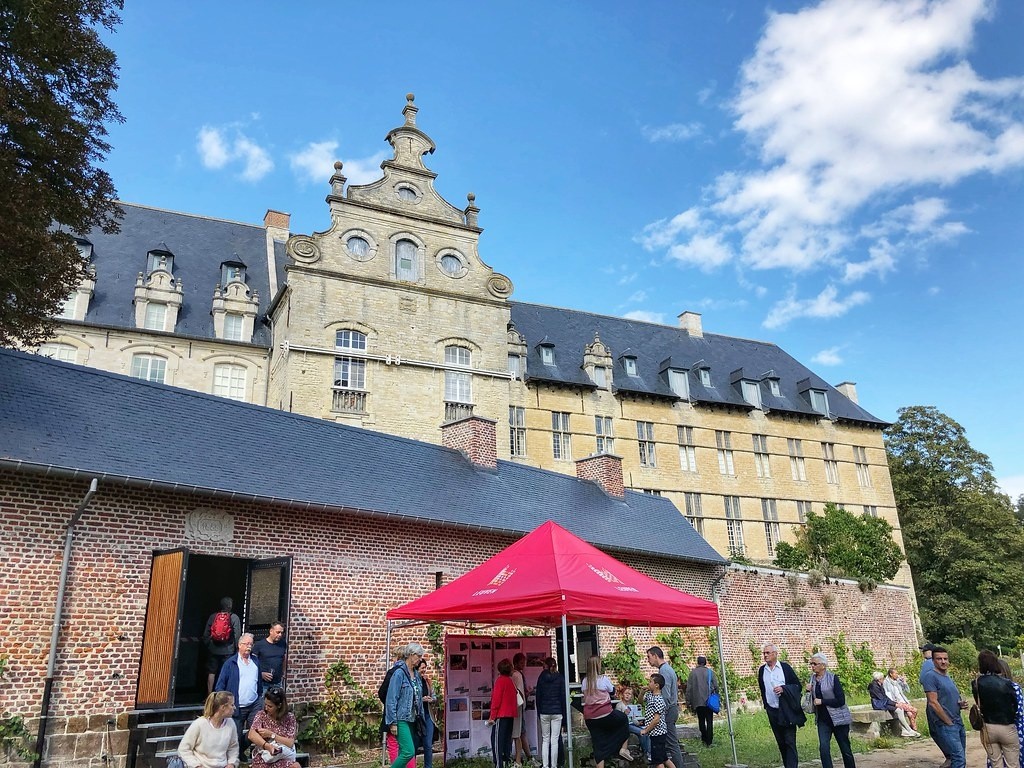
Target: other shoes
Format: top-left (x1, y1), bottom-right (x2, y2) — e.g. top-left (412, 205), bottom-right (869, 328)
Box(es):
top-left (619, 748), bottom-right (634, 762)
top-left (907, 729), bottom-right (921, 738)
top-left (527, 757), bottom-right (542, 767)
top-left (513, 761), bottom-right (522, 768)
top-left (901, 729), bottom-right (915, 738)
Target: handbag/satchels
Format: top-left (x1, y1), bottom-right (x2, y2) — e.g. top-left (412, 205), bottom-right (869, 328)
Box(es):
top-left (969, 678), bottom-right (994, 755)
top-left (509, 676), bottom-right (525, 707)
top-left (800, 673), bottom-right (817, 714)
top-left (252, 739), bottom-right (295, 764)
top-left (707, 693), bottom-right (720, 715)
top-left (415, 714), bottom-right (427, 738)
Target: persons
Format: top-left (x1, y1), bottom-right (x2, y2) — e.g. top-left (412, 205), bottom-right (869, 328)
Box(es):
top-left (638, 673), bottom-right (676, 768)
top-left (248, 687), bottom-right (302, 768)
top-left (251, 621), bottom-right (287, 696)
top-left (685, 657), bottom-right (719, 748)
top-left (918, 644), bottom-right (969, 768)
top-left (647, 646), bottom-right (683, 768)
top-left (805, 653), bottom-right (856, 768)
top-left (378, 642), bottom-right (437, 768)
top-left (615, 688), bottom-right (651, 762)
top-left (202, 597), bottom-right (241, 695)
top-left (487, 652), bottom-right (567, 768)
top-left (758, 643), bottom-right (802, 768)
top-left (215, 633), bottom-right (264, 762)
top-left (581, 656), bottom-right (634, 768)
top-left (177, 691), bottom-right (240, 768)
top-left (870, 668), bottom-right (921, 738)
top-left (971, 645), bottom-right (1024, 768)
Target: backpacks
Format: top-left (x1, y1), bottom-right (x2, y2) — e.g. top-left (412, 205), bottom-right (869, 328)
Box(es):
top-left (210, 610), bottom-right (233, 643)
top-left (378, 664), bottom-right (415, 704)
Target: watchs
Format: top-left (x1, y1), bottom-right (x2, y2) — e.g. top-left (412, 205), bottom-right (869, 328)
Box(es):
top-left (270, 733), bottom-right (277, 740)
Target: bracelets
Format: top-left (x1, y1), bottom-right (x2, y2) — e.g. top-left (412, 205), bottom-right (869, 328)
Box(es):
top-left (263, 741), bottom-right (269, 750)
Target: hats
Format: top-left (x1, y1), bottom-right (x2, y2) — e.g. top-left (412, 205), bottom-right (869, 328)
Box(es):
top-left (918, 644), bottom-right (936, 651)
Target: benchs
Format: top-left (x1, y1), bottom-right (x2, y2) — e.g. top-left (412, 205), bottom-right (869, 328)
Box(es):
top-left (560, 726), bottom-right (703, 768)
top-left (849, 709), bottom-right (908, 740)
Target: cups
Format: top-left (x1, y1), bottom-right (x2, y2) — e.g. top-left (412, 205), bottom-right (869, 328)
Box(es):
top-left (959, 695), bottom-right (966, 708)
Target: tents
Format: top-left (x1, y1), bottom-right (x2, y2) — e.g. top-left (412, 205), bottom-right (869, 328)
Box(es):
top-left (385, 521), bottom-right (738, 768)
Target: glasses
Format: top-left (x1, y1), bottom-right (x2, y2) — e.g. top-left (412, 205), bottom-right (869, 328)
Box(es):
top-left (810, 662), bottom-right (820, 666)
top-left (267, 687), bottom-right (279, 695)
top-left (762, 652), bottom-right (770, 654)
